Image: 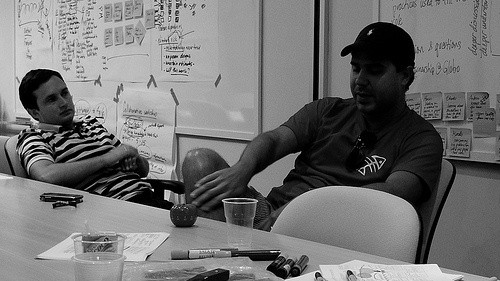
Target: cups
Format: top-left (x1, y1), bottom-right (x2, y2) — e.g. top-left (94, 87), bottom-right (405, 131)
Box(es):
top-left (72, 233), bottom-right (126, 255)
top-left (71, 252), bottom-right (127, 281)
top-left (222, 198), bottom-right (258, 251)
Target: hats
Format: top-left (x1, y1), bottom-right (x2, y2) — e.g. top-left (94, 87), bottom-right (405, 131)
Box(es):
top-left (340, 22), bottom-right (416, 65)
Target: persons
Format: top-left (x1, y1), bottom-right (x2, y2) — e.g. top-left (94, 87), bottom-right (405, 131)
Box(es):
top-left (16, 69), bottom-right (174, 209)
top-left (182, 22), bottom-right (443, 233)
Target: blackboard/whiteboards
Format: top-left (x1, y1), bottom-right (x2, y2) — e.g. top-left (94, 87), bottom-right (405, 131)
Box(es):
top-left (12, 0), bottom-right (263, 142)
top-left (372, 0), bottom-right (500, 164)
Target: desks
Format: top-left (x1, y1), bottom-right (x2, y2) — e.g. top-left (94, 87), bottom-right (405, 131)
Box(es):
top-left (0, 172), bottom-right (493, 281)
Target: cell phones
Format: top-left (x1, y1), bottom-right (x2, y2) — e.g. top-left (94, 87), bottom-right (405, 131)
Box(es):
top-left (40, 193), bottom-right (83, 202)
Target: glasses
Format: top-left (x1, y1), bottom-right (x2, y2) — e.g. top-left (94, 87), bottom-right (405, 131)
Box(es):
top-left (345, 130), bottom-right (377, 172)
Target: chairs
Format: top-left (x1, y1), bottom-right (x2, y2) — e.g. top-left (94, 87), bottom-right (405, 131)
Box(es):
top-left (4, 135), bottom-right (184, 199)
top-left (417, 159), bottom-right (457, 264)
top-left (270, 185), bottom-right (418, 265)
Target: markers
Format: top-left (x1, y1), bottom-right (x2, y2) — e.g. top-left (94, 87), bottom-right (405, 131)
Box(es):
top-left (275, 258), bottom-right (295, 279)
top-left (266, 255), bottom-right (286, 274)
top-left (290, 254), bottom-right (310, 277)
top-left (346, 269), bottom-right (360, 281)
top-left (315, 272), bottom-right (326, 281)
top-left (170, 247), bottom-right (282, 261)
